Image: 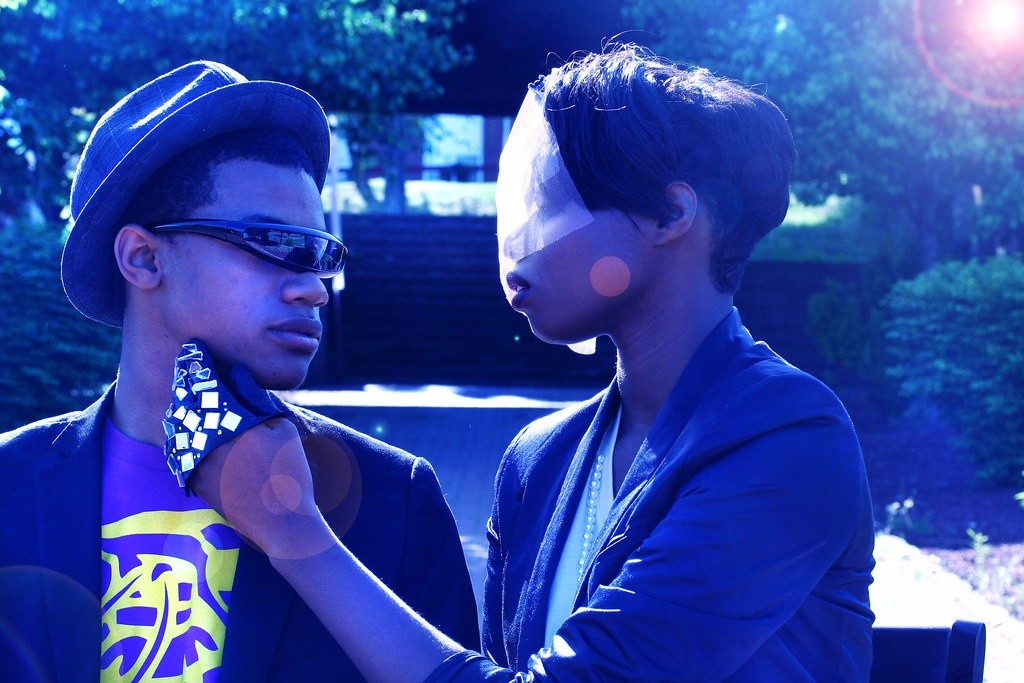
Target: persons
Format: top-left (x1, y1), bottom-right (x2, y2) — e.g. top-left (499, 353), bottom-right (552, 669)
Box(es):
top-left (0, 59), bottom-right (480, 683)
top-left (162, 33), bottom-right (877, 683)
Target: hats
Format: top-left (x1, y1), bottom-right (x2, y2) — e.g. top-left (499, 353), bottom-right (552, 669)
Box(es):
top-left (61, 59), bottom-right (330, 327)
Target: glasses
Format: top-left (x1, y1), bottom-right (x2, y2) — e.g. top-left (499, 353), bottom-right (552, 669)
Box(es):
top-left (150, 219), bottom-right (347, 278)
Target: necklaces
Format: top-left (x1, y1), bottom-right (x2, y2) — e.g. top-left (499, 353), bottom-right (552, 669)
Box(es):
top-left (578, 453), bottom-right (604, 582)
